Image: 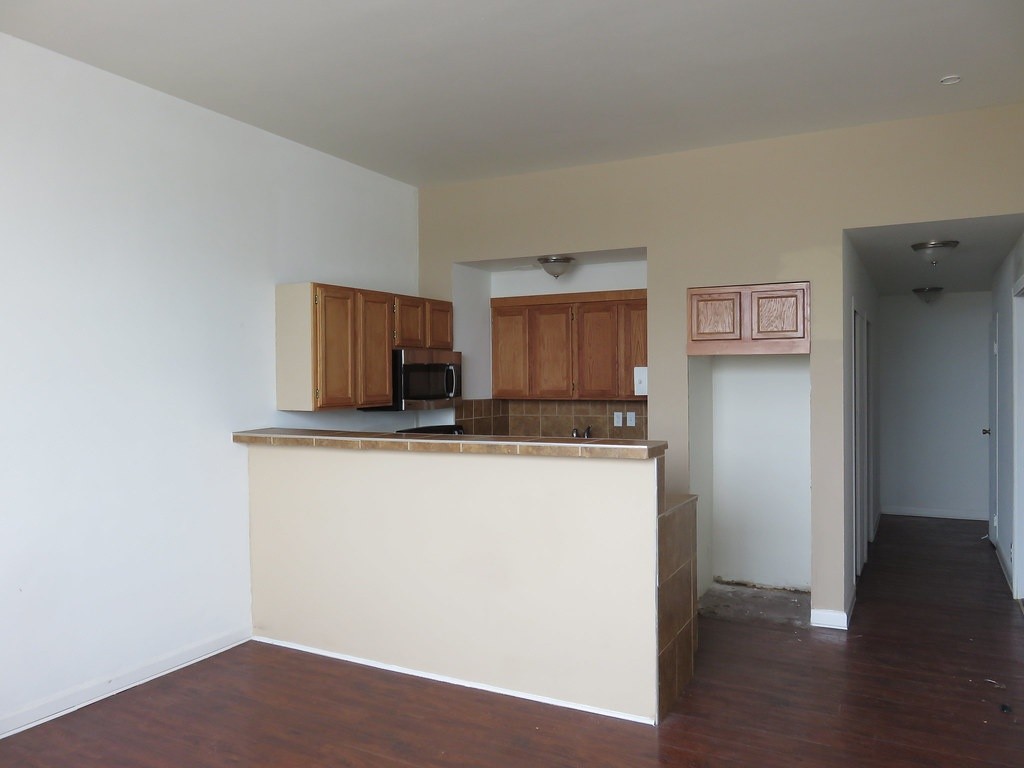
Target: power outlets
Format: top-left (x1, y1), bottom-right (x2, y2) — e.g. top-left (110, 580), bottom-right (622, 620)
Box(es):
top-left (1010, 542), bottom-right (1013, 563)
top-left (613, 411), bottom-right (623, 427)
top-left (626, 412), bottom-right (636, 427)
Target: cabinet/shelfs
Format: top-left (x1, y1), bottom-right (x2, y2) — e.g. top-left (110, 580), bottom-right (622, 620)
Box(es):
top-left (686, 282), bottom-right (811, 359)
top-left (575, 289), bottom-right (647, 401)
top-left (274, 281), bottom-right (394, 411)
top-left (490, 294), bottom-right (576, 400)
top-left (394, 293), bottom-right (453, 351)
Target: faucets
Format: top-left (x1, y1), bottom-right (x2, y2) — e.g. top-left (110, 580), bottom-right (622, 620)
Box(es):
top-left (572, 429), bottom-right (577, 438)
top-left (584, 424), bottom-right (592, 438)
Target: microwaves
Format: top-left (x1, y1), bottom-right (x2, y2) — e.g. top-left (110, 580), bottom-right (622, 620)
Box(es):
top-left (357, 349), bottom-right (462, 412)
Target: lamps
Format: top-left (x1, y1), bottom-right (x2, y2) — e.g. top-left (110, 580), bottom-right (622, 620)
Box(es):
top-left (537, 256), bottom-right (575, 280)
top-left (911, 241), bottom-right (959, 267)
top-left (912, 287), bottom-right (945, 304)
